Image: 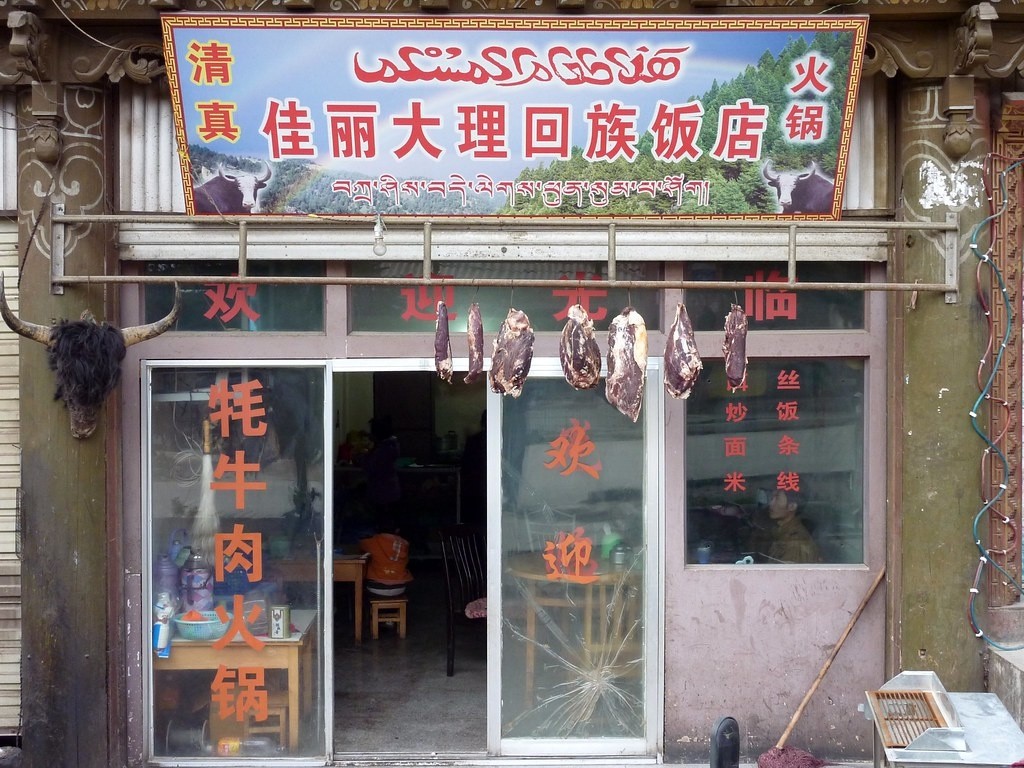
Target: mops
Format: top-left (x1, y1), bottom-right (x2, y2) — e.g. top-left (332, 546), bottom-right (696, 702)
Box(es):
top-left (756, 563), bottom-right (886, 768)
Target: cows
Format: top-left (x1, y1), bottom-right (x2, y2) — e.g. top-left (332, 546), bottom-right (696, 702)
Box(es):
top-left (0, 272), bottom-right (182, 439)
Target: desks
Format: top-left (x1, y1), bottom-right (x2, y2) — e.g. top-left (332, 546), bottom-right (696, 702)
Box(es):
top-left (503, 552), bottom-right (641, 708)
top-left (153, 609), bottom-right (317, 757)
top-left (335, 464), bottom-right (466, 560)
top-left (271, 549), bottom-right (371, 640)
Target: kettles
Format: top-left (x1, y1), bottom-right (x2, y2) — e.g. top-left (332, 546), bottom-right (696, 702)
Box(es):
top-left (609, 542), bottom-right (633, 565)
top-left (153, 527), bottom-right (263, 622)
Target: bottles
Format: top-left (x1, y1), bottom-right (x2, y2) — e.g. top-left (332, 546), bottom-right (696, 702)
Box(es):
top-left (212, 737), bottom-right (284, 757)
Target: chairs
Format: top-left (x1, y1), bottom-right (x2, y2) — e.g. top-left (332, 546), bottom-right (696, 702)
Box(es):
top-left (440, 523), bottom-right (487, 676)
top-left (581, 569), bottom-right (642, 713)
top-left (523, 507), bottom-right (576, 553)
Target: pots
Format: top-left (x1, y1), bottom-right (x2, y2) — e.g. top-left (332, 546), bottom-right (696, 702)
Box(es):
top-left (434, 431), bottom-right (458, 450)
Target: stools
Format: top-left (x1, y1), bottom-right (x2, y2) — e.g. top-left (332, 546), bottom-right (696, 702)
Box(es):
top-left (242, 690), bottom-right (289, 746)
top-left (370, 596), bottom-right (408, 640)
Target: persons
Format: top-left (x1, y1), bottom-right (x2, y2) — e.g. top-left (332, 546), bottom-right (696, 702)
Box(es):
top-left (756, 475), bottom-right (823, 563)
top-left (530, 462), bottom-right (773, 565)
top-left (341, 513), bottom-right (414, 626)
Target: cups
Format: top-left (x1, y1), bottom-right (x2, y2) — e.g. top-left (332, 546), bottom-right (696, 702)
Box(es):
top-left (697, 548), bottom-right (710, 564)
top-left (153, 592), bottom-right (177, 650)
